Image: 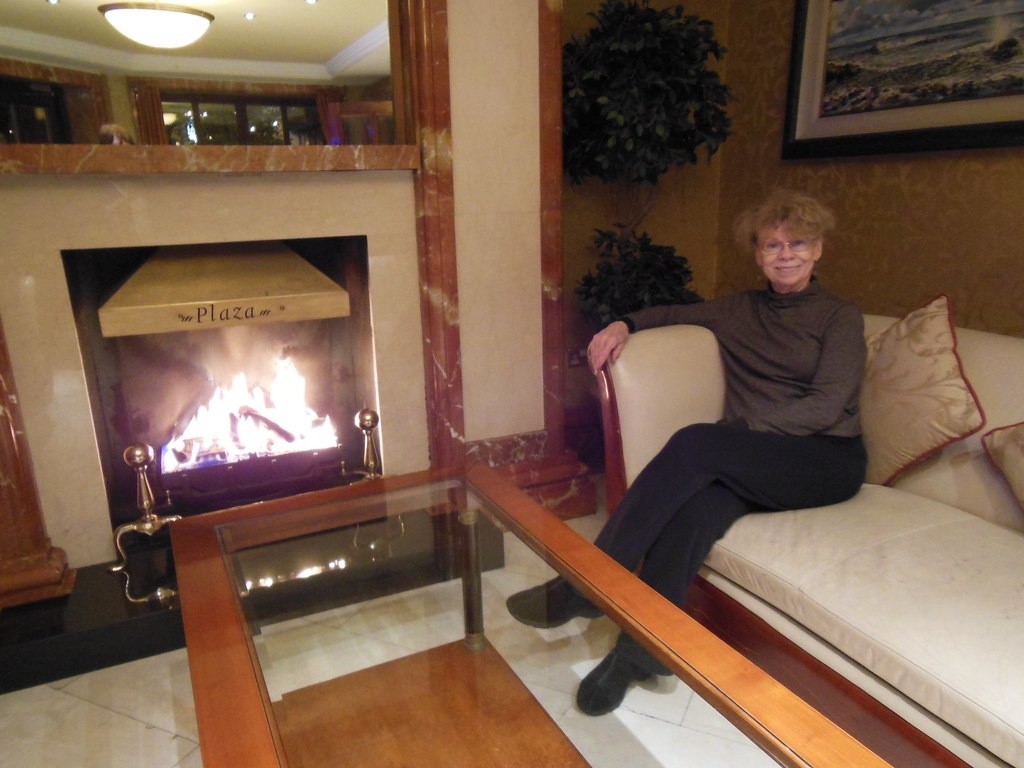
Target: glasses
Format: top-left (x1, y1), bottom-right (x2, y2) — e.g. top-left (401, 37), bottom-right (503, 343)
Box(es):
top-left (756, 236), bottom-right (817, 255)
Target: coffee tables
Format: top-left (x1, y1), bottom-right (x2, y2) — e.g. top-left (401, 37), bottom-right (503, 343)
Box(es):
top-left (165, 459), bottom-right (899, 767)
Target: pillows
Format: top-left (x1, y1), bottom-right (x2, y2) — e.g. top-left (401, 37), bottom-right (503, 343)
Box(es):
top-left (861, 295), bottom-right (988, 487)
top-left (981, 419), bottom-right (1024, 507)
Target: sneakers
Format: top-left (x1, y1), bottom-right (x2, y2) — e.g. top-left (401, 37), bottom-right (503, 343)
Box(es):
top-left (505, 574), bottom-right (607, 629)
top-left (576, 646), bottom-right (652, 716)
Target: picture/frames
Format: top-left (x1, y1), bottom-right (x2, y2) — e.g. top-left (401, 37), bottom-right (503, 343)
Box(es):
top-left (779, 2), bottom-right (1022, 163)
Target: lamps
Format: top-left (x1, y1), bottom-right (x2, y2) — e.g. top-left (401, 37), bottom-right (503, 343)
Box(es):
top-left (93, 2), bottom-right (223, 52)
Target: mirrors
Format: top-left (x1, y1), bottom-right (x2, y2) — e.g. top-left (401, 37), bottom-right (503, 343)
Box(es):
top-left (0, 2), bottom-right (403, 151)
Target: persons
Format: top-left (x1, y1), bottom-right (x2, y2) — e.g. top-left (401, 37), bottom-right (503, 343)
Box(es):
top-left (505, 190), bottom-right (866, 716)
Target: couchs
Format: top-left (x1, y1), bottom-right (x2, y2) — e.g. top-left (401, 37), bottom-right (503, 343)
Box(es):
top-left (584, 314), bottom-right (1023, 768)
